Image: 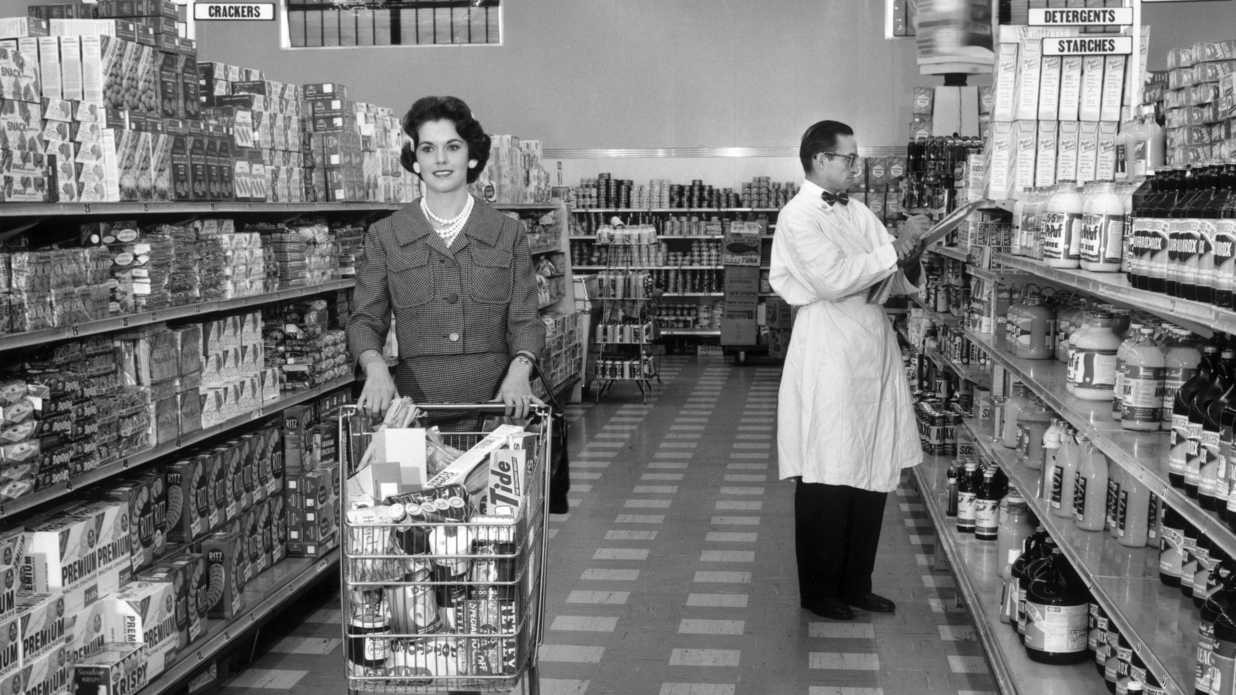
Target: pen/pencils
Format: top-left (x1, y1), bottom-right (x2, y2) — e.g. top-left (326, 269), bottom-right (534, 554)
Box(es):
top-left (902, 212), bottom-right (910, 217)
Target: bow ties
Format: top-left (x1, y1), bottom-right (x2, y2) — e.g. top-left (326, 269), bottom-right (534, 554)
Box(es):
top-left (821, 191), bottom-right (849, 207)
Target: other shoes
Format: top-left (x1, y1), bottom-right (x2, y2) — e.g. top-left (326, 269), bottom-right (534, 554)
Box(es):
top-left (848, 592), bottom-right (895, 612)
top-left (801, 599), bottom-right (854, 619)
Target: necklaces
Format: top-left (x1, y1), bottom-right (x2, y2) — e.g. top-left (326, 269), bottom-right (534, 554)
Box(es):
top-left (420, 192), bottom-right (475, 248)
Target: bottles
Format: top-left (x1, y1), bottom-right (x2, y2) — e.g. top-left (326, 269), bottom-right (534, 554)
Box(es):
top-left (907, 101), bottom-right (1234, 694)
top-left (390, 505), bottom-right (435, 567)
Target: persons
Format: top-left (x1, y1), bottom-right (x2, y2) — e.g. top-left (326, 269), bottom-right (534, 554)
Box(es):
top-left (768, 120), bottom-right (934, 621)
top-left (344, 97), bottom-right (546, 449)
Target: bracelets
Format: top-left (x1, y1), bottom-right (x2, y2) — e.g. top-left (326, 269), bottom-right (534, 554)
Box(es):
top-left (508, 356), bottom-right (534, 370)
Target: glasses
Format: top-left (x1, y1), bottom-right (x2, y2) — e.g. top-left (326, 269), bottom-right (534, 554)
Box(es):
top-left (814, 153), bottom-right (859, 169)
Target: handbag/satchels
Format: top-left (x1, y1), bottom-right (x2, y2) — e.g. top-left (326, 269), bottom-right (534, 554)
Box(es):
top-left (476, 407), bottom-right (570, 515)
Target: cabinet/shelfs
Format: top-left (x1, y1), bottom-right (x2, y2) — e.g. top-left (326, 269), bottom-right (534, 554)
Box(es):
top-left (590, 242), bottom-right (657, 405)
top-left (0, 195), bottom-right (575, 695)
top-left (570, 208), bottom-right (783, 335)
top-left (912, 245), bottom-right (1236, 695)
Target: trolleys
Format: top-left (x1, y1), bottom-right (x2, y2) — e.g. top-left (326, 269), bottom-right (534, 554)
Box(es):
top-left (337, 398), bottom-right (554, 695)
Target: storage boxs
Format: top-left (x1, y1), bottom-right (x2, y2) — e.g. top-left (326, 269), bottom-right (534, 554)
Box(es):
top-left (0, 0), bottom-right (581, 695)
top-left (698, 222), bottom-right (794, 358)
top-left (953, 25), bottom-right (1150, 252)
